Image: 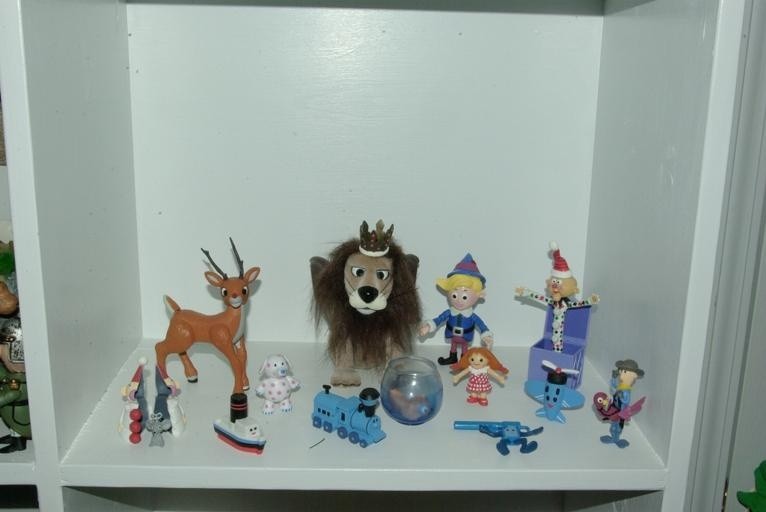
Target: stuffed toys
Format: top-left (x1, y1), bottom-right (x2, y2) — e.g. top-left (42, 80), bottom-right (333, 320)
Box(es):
top-left (308, 218), bottom-right (424, 387)
top-left (117, 356), bottom-right (185, 447)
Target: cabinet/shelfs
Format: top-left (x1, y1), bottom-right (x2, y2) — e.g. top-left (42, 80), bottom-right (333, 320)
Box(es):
top-left (0, 0), bottom-right (766, 512)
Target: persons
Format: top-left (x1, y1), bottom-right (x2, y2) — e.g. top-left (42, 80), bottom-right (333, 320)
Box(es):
top-left (450, 347), bottom-right (510, 405)
top-left (418, 252), bottom-right (494, 367)
top-left (512, 241), bottom-right (601, 391)
top-left (591, 358), bottom-right (647, 448)
